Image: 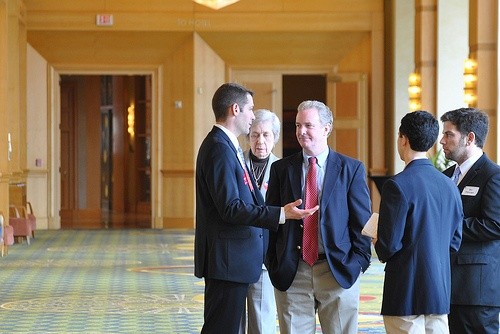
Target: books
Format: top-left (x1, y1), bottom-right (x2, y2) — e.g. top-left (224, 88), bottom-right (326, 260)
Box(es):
top-left (360, 211), bottom-right (379, 240)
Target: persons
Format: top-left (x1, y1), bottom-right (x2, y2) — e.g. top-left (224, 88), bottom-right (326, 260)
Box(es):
top-left (192, 82), bottom-right (320, 334)
top-left (266, 100), bottom-right (372, 334)
top-left (237, 108), bottom-right (283, 334)
top-left (438, 107), bottom-right (500, 334)
top-left (372, 110), bottom-right (464, 334)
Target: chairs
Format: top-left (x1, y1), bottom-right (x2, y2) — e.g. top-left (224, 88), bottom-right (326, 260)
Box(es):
top-left (0, 202), bottom-right (36, 257)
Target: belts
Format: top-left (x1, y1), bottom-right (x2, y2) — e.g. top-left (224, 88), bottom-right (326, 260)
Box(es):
top-left (300, 253), bottom-right (328, 264)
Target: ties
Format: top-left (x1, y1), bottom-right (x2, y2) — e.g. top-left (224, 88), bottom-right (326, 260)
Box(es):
top-left (302, 157), bottom-right (319, 267)
top-left (448, 166), bottom-right (462, 186)
top-left (237, 145), bottom-right (250, 170)
